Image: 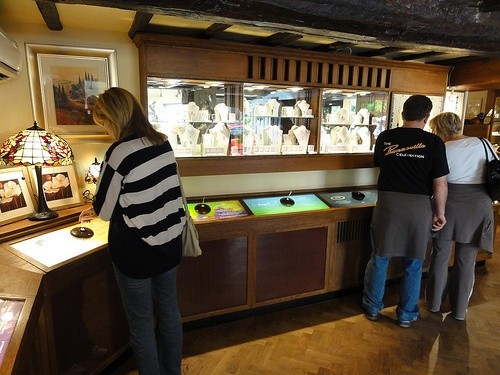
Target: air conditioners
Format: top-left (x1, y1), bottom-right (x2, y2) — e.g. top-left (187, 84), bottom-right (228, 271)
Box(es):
top-left (0, 27), bottom-right (23, 84)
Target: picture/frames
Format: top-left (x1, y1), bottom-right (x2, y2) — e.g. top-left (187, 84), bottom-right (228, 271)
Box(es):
top-left (27, 164), bottom-right (85, 211)
top-left (0, 166), bottom-right (38, 227)
top-left (24, 41), bottom-right (120, 144)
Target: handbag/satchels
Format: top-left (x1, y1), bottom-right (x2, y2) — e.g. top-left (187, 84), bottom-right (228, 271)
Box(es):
top-left (182, 210), bottom-right (202, 257)
top-left (480, 137), bottom-right (500, 202)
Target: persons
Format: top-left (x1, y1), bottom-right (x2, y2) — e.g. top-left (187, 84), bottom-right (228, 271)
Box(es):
top-left (430, 112), bottom-right (500, 321)
top-left (360, 94), bottom-right (450, 328)
top-left (92, 86), bottom-right (187, 375)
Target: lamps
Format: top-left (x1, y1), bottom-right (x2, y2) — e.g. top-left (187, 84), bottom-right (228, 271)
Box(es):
top-left (0, 121), bottom-right (74, 221)
top-left (84, 158), bottom-right (101, 184)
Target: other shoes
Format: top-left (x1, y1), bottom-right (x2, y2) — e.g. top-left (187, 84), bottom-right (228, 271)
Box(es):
top-left (399, 311), bottom-right (421, 327)
top-left (363, 311), bottom-right (381, 320)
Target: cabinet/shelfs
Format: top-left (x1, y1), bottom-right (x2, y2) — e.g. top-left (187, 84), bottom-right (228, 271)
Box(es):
top-left (146, 79), bottom-right (467, 158)
